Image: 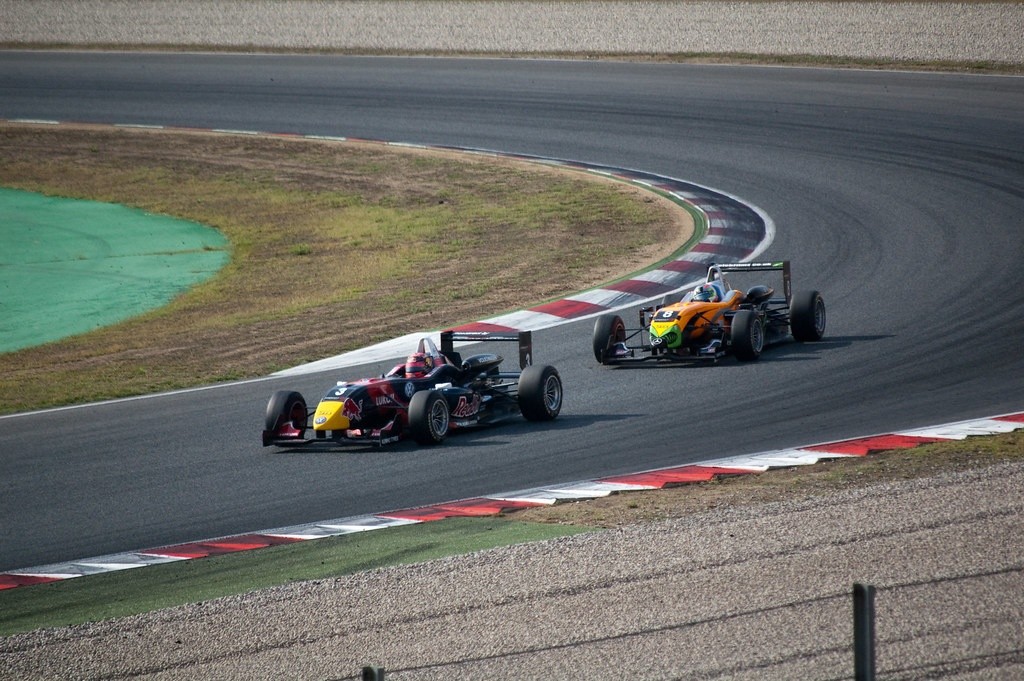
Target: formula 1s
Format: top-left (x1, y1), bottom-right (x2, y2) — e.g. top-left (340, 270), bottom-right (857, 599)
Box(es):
top-left (592, 261), bottom-right (826, 366)
top-left (262, 322), bottom-right (563, 448)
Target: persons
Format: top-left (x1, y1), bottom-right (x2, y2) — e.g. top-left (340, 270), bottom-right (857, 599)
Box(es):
top-left (693, 286), bottom-right (718, 301)
top-left (405, 353), bottom-right (435, 379)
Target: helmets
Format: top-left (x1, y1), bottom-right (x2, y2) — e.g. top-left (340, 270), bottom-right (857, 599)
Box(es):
top-left (405, 353), bottom-right (434, 378)
top-left (692, 285), bottom-right (718, 302)
